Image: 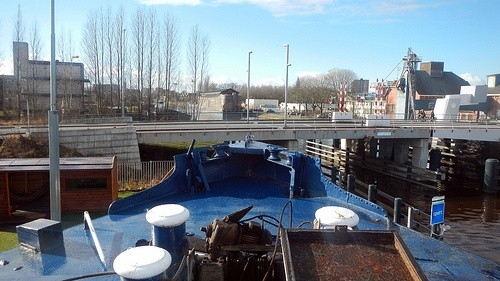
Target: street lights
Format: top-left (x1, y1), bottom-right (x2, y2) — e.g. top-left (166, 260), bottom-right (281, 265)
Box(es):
top-left (70, 56), bottom-right (79, 108)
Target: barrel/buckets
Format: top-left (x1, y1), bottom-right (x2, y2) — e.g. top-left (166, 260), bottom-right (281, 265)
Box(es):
top-left (313, 206), bottom-right (360, 230)
top-left (146, 204), bottom-right (190, 281)
top-left (113, 245), bottom-right (172, 281)
